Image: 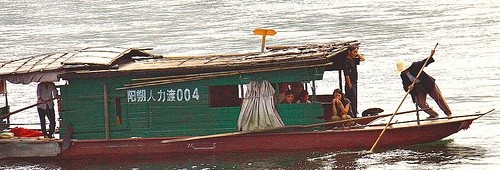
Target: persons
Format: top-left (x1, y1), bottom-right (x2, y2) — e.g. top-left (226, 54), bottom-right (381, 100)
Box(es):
top-left (292, 83), bottom-right (304, 104)
top-left (297, 90), bottom-right (311, 104)
top-left (36, 81), bottom-right (58, 137)
top-left (330, 88), bottom-right (354, 127)
top-left (344, 45), bottom-right (365, 117)
top-left (275, 86), bottom-right (287, 103)
top-left (394, 49), bottom-right (452, 119)
top-left (280, 90), bottom-right (294, 104)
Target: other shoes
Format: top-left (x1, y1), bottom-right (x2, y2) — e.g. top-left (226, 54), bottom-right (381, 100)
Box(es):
top-left (49, 133), bottom-right (55, 138)
top-left (44, 134), bottom-right (48, 138)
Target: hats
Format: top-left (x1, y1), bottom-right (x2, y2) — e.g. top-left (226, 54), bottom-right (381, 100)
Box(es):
top-left (395, 61), bottom-right (412, 74)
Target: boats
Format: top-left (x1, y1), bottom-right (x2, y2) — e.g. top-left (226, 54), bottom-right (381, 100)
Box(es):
top-left (0, 28), bottom-right (496, 160)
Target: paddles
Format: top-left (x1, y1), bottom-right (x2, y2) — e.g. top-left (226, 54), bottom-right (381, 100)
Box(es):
top-left (369, 42), bottom-right (439, 151)
top-left (0, 98), bottom-right (56, 120)
top-left (160, 107), bottom-right (432, 144)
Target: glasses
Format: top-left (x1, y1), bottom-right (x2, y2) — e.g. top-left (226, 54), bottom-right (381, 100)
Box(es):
top-left (352, 51), bottom-right (358, 53)
